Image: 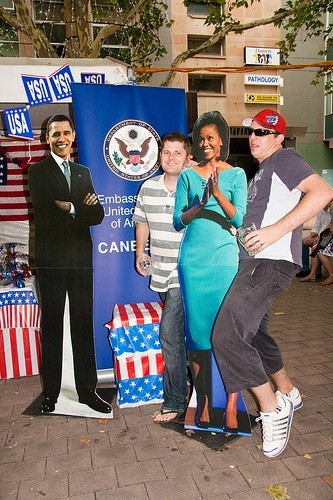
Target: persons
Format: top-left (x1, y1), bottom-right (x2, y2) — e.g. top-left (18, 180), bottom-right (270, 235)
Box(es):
top-left (296, 202), bottom-right (332, 278)
top-left (211, 109), bottom-right (333, 458)
top-left (299, 196), bottom-right (333, 285)
top-left (131, 131), bottom-right (193, 424)
top-left (29, 114), bottom-right (112, 413)
top-left (173, 108), bottom-right (247, 432)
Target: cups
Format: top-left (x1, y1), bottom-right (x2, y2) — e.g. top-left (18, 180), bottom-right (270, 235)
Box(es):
top-left (138, 256), bottom-right (157, 276)
top-left (237, 222), bottom-right (264, 257)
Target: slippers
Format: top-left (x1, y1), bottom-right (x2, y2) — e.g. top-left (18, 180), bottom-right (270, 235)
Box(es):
top-left (153, 409), bottom-right (181, 423)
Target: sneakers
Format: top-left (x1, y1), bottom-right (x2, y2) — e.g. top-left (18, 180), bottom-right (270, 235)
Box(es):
top-left (254, 396), bottom-right (294, 457)
top-left (283, 387), bottom-right (303, 411)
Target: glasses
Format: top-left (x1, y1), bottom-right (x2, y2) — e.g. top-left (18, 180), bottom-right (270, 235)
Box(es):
top-left (246, 128), bottom-right (279, 137)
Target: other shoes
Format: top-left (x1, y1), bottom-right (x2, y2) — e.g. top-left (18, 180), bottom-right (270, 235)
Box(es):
top-left (223, 412), bottom-right (239, 433)
top-left (195, 411), bottom-right (210, 429)
top-left (299, 274), bottom-right (315, 282)
top-left (320, 276), bottom-right (333, 286)
top-left (296, 269), bottom-right (311, 277)
top-left (316, 272), bottom-right (322, 279)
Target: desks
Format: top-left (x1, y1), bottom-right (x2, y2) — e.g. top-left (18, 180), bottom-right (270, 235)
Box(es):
top-left (0, 276), bottom-right (41, 380)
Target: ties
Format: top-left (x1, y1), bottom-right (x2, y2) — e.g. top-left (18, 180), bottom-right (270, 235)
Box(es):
top-left (62, 161), bottom-right (70, 194)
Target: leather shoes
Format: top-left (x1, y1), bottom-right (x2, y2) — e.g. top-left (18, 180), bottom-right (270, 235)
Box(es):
top-left (79, 390), bottom-right (111, 413)
top-left (41, 396), bottom-right (57, 412)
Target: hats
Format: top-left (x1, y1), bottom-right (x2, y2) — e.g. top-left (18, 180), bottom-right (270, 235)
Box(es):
top-left (242, 109), bottom-right (286, 136)
